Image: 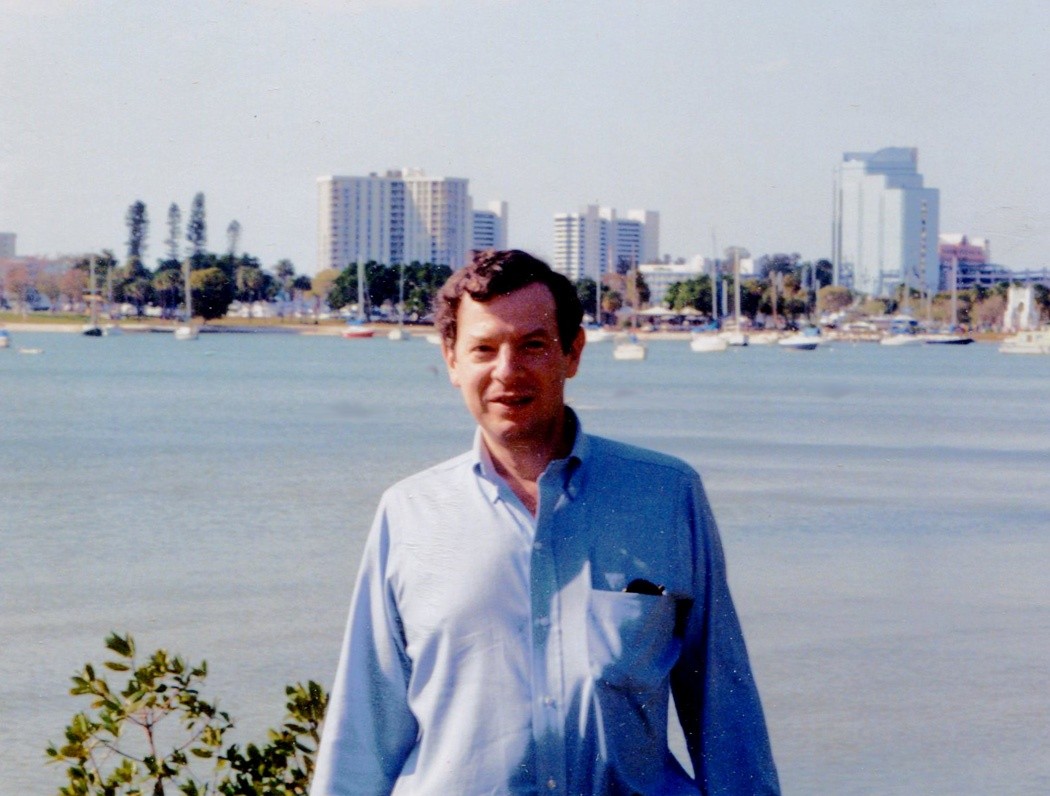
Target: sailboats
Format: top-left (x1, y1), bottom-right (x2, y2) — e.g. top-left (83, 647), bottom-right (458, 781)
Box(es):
top-left (82, 255), bottom-right (104, 337)
top-left (385, 259), bottom-right (410, 341)
top-left (344, 252), bottom-right (377, 339)
top-left (100, 263), bottom-right (124, 337)
top-left (878, 256), bottom-right (978, 348)
top-left (611, 244), bottom-right (648, 363)
top-left (175, 262), bottom-right (200, 337)
top-left (581, 265), bottom-right (615, 342)
top-left (687, 250), bottom-right (822, 355)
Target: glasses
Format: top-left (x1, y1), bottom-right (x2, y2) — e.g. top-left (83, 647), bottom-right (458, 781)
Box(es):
top-left (621, 577), bottom-right (667, 597)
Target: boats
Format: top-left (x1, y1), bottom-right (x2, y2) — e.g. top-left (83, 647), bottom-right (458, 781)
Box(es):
top-left (996, 327), bottom-right (1050, 352)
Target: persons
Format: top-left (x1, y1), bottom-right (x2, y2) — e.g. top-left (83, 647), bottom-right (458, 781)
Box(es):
top-left (307, 246), bottom-right (784, 796)
